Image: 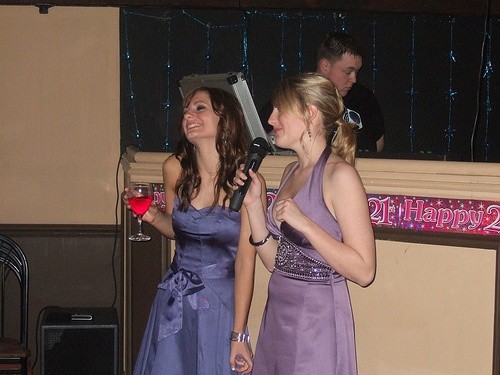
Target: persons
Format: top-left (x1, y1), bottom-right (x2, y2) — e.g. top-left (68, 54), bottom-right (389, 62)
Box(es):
top-left (232, 72), bottom-right (377, 375)
top-left (124, 86), bottom-right (267, 375)
top-left (314, 31), bottom-right (384, 158)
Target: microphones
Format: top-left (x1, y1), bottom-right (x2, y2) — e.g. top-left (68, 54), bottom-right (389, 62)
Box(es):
top-left (229, 137), bottom-right (268, 211)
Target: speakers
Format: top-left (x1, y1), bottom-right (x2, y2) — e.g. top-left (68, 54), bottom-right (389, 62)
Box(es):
top-left (40, 307), bottom-right (118, 375)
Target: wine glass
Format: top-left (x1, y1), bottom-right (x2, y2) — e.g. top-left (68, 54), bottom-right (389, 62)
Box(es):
top-left (128, 183), bottom-right (154, 241)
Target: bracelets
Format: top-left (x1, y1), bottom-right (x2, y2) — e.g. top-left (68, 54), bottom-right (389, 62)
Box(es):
top-left (230, 331), bottom-right (250, 344)
top-left (249, 233), bottom-right (272, 246)
